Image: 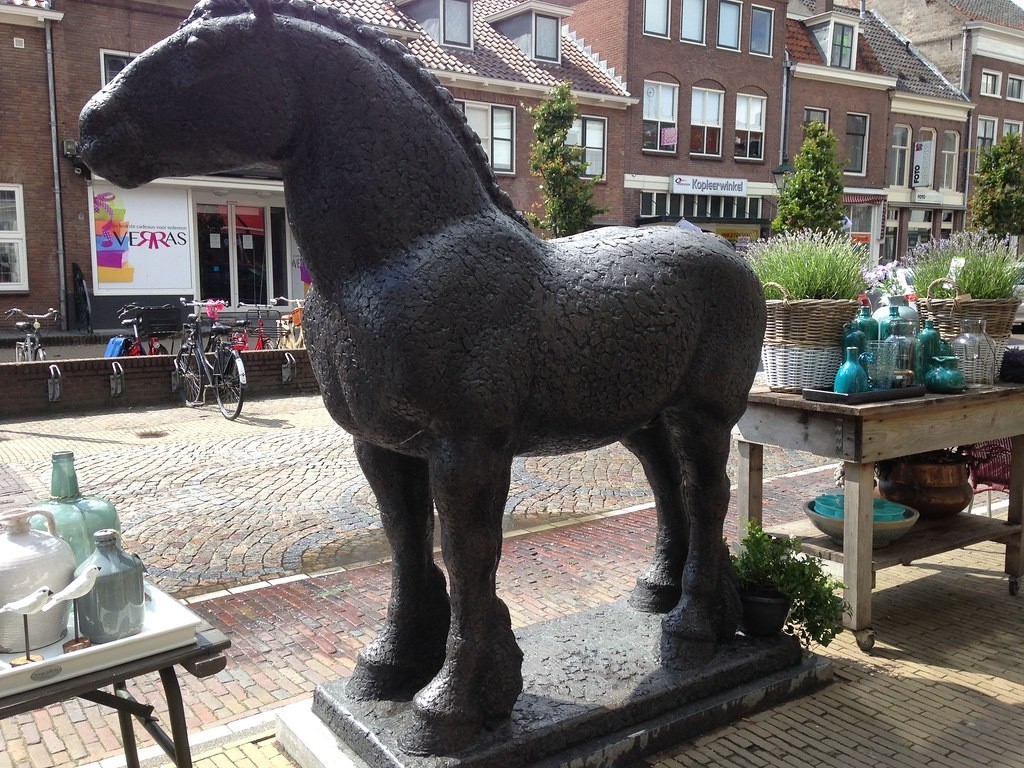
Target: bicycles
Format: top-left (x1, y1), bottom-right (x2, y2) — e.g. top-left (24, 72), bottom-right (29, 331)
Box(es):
top-left (269, 296), bottom-right (307, 350)
top-left (4, 307), bottom-right (61, 361)
top-left (180, 299), bottom-right (217, 354)
top-left (175, 298), bottom-right (247, 420)
top-left (227, 301), bottom-right (275, 352)
top-left (111, 301), bottom-right (174, 366)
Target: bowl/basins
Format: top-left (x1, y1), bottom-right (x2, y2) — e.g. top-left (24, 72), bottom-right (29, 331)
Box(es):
top-left (802, 499), bottom-right (920, 548)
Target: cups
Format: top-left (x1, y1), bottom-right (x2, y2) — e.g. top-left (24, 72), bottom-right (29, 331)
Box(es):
top-left (857, 340), bottom-right (898, 391)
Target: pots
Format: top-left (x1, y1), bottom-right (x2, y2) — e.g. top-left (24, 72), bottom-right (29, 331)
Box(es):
top-left (872, 451), bottom-right (980, 522)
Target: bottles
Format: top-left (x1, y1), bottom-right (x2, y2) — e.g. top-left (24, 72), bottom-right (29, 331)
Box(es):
top-left (833, 305), bottom-right (997, 394)
top-left (25, 451), bottom-right (121, 612)
top-left (74, 529), bottom-right (144, 644)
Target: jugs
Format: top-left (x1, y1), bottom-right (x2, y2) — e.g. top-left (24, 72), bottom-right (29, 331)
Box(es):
top-left (0, 508), bottom-right (77, 653)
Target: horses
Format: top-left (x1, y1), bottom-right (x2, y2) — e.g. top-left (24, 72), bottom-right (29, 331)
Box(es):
top-left (76, 1), bottom-right (768, 759)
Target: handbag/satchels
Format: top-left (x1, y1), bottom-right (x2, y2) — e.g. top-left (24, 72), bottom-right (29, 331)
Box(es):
top-left (103, 337), bottom-right (133, 357)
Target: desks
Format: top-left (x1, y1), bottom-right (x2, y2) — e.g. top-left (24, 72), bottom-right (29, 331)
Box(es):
top-left (731, 381), bottom-right (1024, 650)
top-left (0, 572), bottom-right (231, 768)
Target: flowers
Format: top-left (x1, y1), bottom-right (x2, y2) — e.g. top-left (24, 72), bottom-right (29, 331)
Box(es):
top-left (862, 229), bottom-right (1024, 300)
top-left (740, 227), bottom-right (883, 300)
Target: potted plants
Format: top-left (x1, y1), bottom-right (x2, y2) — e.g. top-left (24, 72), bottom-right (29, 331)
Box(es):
top-left (723, 516), bottom-right (854, 658)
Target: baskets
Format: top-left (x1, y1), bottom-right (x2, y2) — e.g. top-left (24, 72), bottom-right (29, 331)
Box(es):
top-left (291, 307), bottom-right (304, 326)
top-left (760, 282), bottom-right (864, 393)
top-left (914, 278), bottom-right (1022, 384)
